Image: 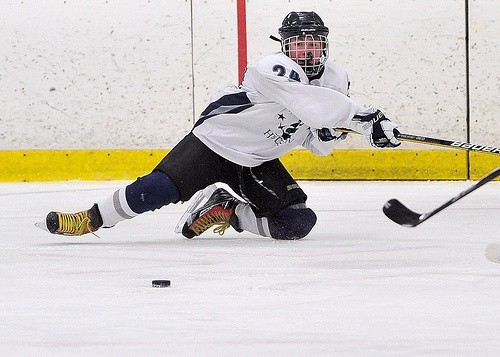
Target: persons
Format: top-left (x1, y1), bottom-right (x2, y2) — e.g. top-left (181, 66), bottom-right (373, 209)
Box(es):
top-left (33, 11), bottom-right (403, 242)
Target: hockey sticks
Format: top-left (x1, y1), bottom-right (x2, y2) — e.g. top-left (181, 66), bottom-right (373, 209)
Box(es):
top-left (383, 167), bottom-right (500, 227)
top-left (333, 127), bottom-right (500, 154)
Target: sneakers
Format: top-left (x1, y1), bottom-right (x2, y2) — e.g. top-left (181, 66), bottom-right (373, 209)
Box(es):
top-left (174, 184), bottom-right (246, 240)
top-left (34, 203), bottom-right (116, 239)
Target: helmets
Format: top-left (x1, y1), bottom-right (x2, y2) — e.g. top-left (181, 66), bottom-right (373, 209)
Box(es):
top-left (278, 11), bottom-right (329, 57)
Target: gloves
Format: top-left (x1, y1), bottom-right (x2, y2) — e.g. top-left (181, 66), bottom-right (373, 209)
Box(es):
top-left (315, 128), bottom-right (348, 143)
top-left (352, 105), bottom-right (401, 150)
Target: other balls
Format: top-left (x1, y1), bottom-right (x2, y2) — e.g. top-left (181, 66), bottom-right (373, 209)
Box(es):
top-left (152, 280), bottom-right (170, 288)
